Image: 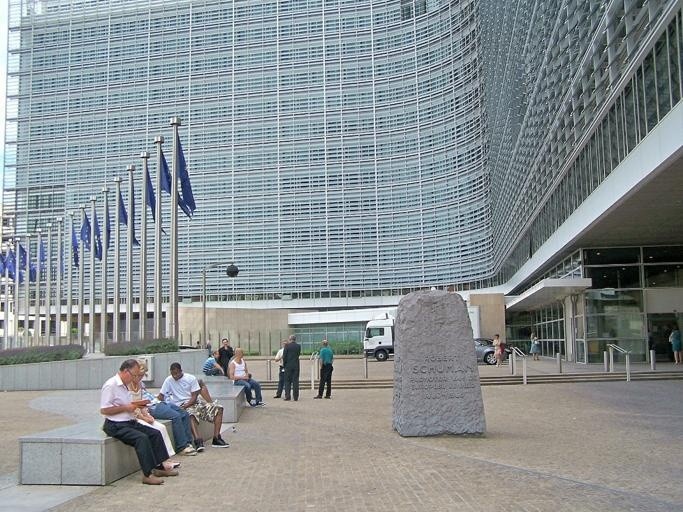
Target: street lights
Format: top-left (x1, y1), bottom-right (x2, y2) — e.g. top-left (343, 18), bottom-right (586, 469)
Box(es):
top-left (201, 262), bottom-right (238, 350)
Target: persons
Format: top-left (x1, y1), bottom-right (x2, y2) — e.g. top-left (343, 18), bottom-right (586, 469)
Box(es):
top-left (529, 333), bottom-right (541, 361)
top-left (99, 338), bottom-right (265, 485)
top-left (282, 335), bottom-right (301, 401)
top-left (313, 340), bottom-right (333, 399)
top-left (273, 339), bottom-right (287, 399)
top-left (493, 335), bottom-right (503, 368)
top-left (669, 325), bottom-right (682, 364)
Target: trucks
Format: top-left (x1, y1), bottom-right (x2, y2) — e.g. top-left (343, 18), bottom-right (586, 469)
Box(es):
top-left (362, 319), bottom-right (395, 361)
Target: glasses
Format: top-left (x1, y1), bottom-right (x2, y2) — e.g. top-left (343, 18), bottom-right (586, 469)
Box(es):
top-left (128, 370), bottom-right (138, 378)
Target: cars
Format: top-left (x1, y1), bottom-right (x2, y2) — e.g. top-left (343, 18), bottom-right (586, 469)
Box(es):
top-left (473, 341), bottom-right (505, 365)
top-left (475, 338), bottom-right (511, 359)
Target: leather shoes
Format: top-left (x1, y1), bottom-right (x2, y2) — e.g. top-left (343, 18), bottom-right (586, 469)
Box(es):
top-left (314, 395), bottom-right (322, 399)
top-left (324, 396), bottom-right (330, 399)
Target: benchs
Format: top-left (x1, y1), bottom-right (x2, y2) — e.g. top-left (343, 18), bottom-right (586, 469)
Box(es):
top-left (16, 375), bottom-right (246, 486)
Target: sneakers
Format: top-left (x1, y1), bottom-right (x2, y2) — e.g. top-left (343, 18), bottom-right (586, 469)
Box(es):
top-left (143, 459), bottom-right (182, 485)
top-left (178, 435), bottom-right (230, 456)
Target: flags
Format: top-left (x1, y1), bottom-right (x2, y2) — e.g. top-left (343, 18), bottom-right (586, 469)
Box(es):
top-left (0, 134), bottom-right (196, 283)
